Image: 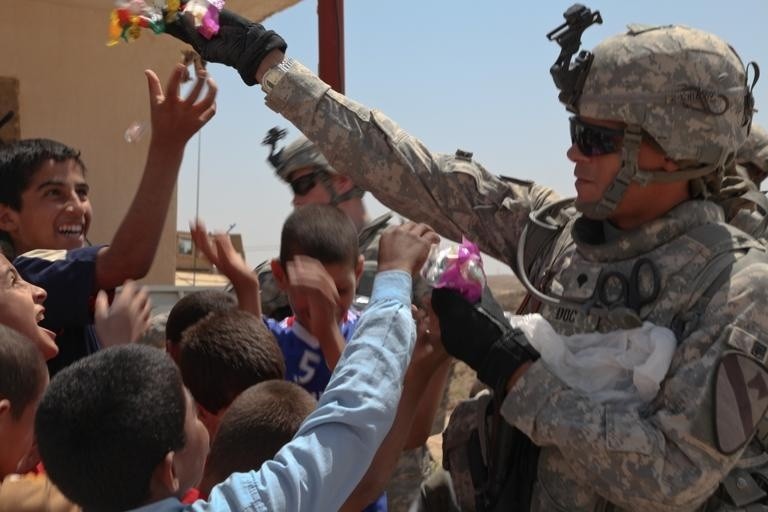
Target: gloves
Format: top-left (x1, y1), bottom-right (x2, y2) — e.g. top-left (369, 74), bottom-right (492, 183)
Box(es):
top-left (430, 268), bottom-right (539, 387)
top-left (143, 1), bottom-right (288, 87)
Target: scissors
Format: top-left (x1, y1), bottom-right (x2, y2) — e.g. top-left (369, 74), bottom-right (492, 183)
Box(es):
top-left (597, 258), bottom-right (661, 317)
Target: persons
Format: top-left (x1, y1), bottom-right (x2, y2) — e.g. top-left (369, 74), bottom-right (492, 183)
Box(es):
top-left (717, 122), bottom-right (767, 247)
top-left (0, 60), bottom-right (463, 512)
top-left (141, 0), bottom-right (767, 511)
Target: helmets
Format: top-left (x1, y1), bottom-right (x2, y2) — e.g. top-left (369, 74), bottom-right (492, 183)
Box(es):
top-left (575, 24), bottom-right (748, 166)
top-left (275, 134), bottom-right (342, 180)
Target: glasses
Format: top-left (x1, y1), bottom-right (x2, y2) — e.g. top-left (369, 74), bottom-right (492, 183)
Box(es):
top-left (290, 170), bottom-right (319, 195)
top-left (569, 115), bottom-right (641, 157)
